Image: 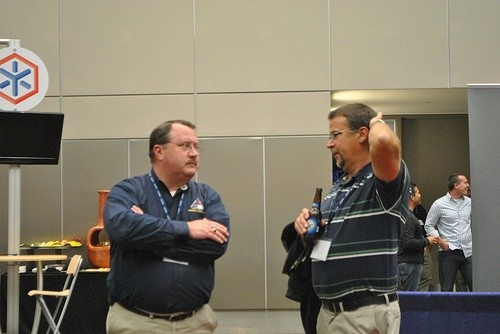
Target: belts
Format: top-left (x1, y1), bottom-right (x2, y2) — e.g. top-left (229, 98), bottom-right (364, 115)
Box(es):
top-left (322, 291), bottom-right (397, 313)
top-left (113, 298), bottom-right (198, 321)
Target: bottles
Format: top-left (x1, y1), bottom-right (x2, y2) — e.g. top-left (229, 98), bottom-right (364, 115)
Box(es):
top-left (302, 187), bottom-right (322, 242)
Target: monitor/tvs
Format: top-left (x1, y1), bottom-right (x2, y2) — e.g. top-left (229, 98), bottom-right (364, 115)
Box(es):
top-left (0, 110), bottom-right (64, 165)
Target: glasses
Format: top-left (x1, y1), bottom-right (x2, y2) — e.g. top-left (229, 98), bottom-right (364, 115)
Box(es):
top-left (162, 142), bottom-right (200, 153)
top-left (329, 128), bottom-right (359, 140)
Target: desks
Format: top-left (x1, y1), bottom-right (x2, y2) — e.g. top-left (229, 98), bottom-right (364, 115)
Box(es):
top-left (0, 254), bottom-right (67, 334)
top-left (0, 268), bottom-right (109, 334)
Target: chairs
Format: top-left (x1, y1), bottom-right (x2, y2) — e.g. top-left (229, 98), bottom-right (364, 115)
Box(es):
top-left (27, 255), bottom-right (83, 334)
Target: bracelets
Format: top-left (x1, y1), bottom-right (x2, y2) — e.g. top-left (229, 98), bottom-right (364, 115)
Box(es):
top-left (369, 119), bottom-right (385, 128)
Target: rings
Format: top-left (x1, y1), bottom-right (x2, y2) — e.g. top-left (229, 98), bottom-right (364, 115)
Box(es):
top-left (435, 242), bottom-right (436, 244)
top-left (213, 228), bottom-right (216, 233)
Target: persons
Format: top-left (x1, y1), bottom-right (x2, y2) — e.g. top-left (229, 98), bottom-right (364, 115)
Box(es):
top-left (103, 119), bottom-right (231, 334)
top-left (424, 173), bottom-right (473, 291)
top-left (294, 103), bottom-right (411, 334)
top-left (397, 182), bottom-right (437, 291)
